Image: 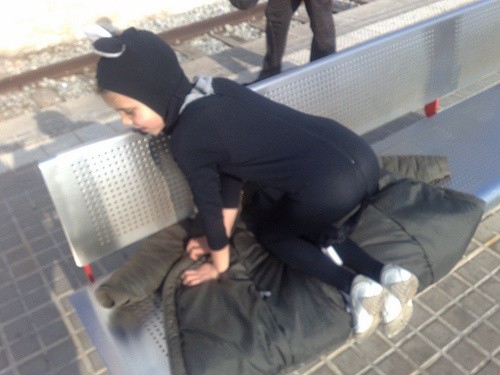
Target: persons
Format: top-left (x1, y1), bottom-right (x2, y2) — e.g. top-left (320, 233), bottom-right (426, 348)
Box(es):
top-left (89, 15), bottom-right (419, 344)
top-left (241, 0), bottom-right (337, 85)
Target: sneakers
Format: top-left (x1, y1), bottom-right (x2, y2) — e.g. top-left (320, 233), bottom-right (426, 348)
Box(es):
top-left (381, 263), bottom-right (418, 339)
top-left (345, 275), bottom-right (390, 346)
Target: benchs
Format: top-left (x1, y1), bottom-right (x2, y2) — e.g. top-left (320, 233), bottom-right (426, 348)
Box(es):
top-left (38, 0), bottom-right (498, 375)
